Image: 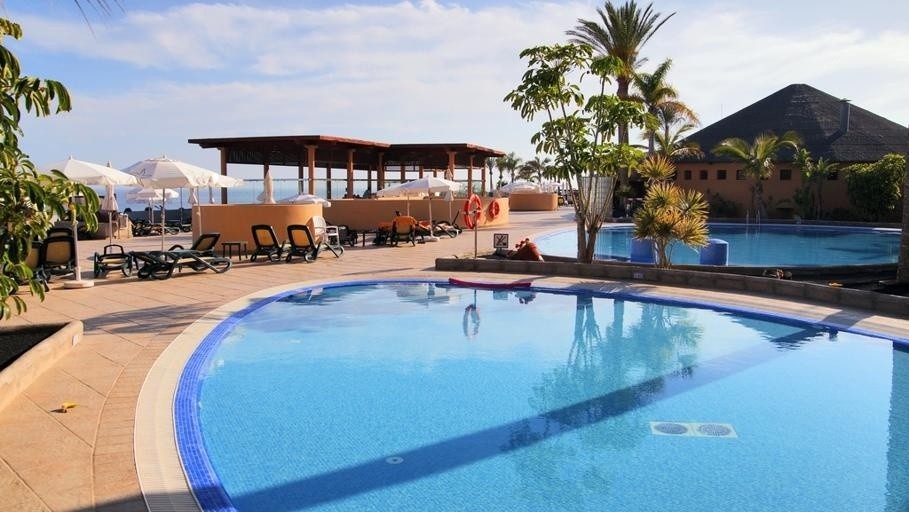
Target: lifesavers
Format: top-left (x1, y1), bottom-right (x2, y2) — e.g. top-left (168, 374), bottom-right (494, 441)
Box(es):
top-left (490, 201), bottom-right (500, 220)
top-left (463, 193), bottom-right (482, 229)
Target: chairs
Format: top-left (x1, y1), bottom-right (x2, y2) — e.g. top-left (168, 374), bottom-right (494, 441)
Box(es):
top-left (362, 212), bottom-right (463, 248)
top-left (17, 226), bottom-right (74, 279)
top-left (96, 215), bottom-right (360, 280)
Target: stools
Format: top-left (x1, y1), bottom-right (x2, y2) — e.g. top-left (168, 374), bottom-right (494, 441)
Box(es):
top-left (698, 239), bottom-right (729, 265)
top-left (629, 235), bottom-right (658, 264)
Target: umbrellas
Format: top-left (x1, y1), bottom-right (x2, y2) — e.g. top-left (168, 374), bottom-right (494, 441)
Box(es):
top-left (261, 168), bottom-right (276, 204)
top-left (277, 192), bottom-right (331, 209)
top-left (41, 155), bottom-right (243, 281)
top-left (499, 181), bottom-right (569, 194)
top-left (376, 167), bottom-right (463, 237)
